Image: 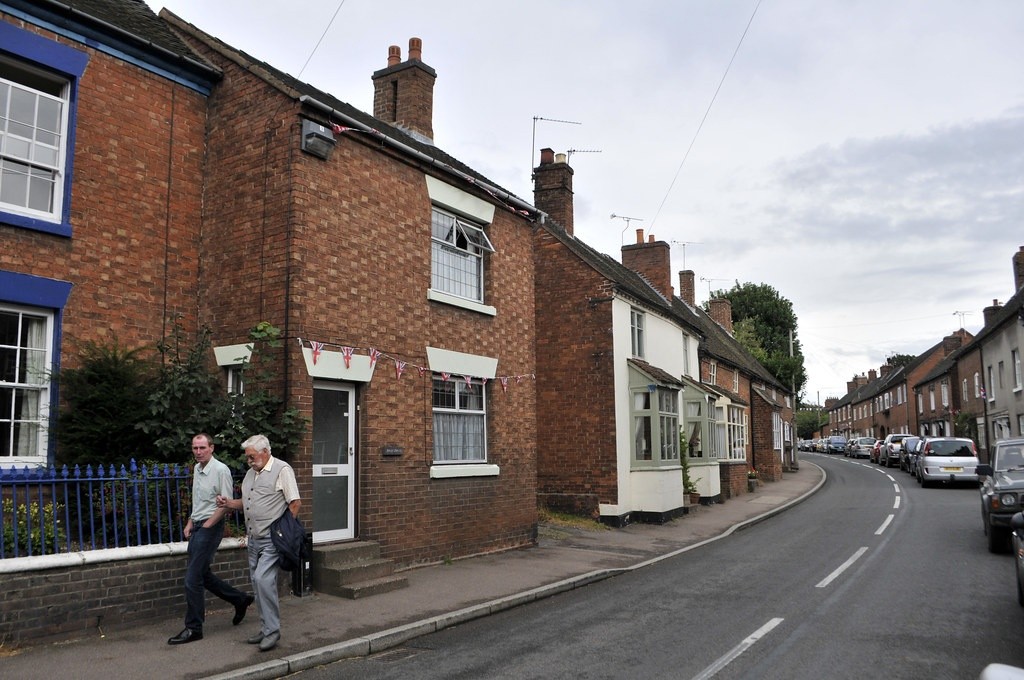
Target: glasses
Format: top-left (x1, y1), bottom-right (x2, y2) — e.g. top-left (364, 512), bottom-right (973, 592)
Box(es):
top-left (246, 452), bottom-right (261, 461)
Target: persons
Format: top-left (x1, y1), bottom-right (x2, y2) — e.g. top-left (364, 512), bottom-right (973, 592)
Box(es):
top-left (215, 433), bottom-right (303, 650)
top-left (167, 433), bottom-right (255, 644)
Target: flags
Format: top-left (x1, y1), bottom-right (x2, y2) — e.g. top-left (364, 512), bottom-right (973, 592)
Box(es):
top-left (980, 382), bottom-right (988, 399)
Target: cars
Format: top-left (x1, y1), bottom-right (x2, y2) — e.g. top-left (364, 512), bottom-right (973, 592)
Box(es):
top-left (798, 434), bottom-right (924, 476)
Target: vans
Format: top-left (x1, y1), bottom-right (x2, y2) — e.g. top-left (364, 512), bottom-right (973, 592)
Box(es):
top-left (913, 437), bottom-right (980, 489)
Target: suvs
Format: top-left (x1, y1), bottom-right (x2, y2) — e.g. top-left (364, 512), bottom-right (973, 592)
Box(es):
top-left (975, 437), bottom-right (1024, 553)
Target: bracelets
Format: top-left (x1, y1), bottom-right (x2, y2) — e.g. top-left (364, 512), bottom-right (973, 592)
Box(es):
top-left (188, 517), bottom-right (191, 520)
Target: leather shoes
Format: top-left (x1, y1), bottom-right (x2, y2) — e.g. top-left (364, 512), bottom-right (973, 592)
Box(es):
top-left (248, 631), bottom-right (264, 642)
top-left (232, 595), bottom-right (257, 625)
top-left (168, 627), bottom-right (203, 644)
top-left (259, 631), bottom-right (281, 650)
